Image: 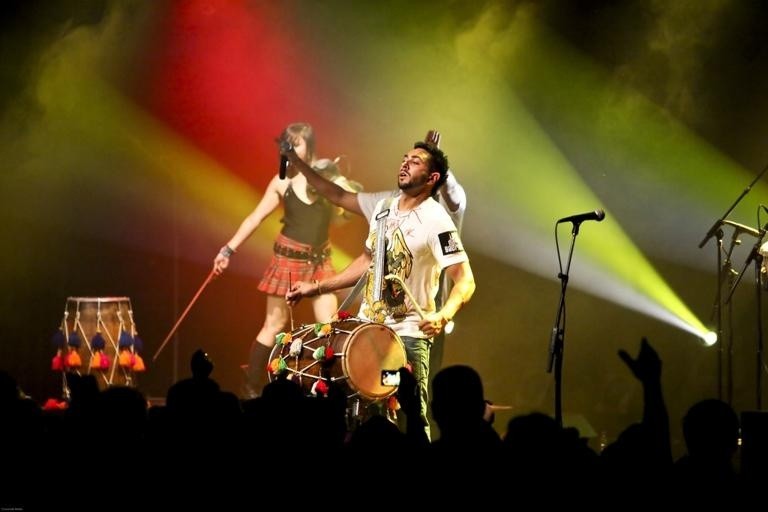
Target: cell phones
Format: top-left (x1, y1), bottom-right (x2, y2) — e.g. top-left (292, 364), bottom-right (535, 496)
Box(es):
top-left (382, 370), bottom-right (401, 386)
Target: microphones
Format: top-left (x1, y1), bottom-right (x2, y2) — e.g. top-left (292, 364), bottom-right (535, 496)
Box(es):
top-left (558, 208), bottom-right (606, 223)
top-left (280, 141), bottom-right (290, 180)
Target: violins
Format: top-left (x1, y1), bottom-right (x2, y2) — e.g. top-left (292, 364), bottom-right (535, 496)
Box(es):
top-left (307, 158), bottom-right (362, 215)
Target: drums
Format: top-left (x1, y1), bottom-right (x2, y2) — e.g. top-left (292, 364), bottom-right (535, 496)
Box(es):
top-left (267, 320), bottom-right (408, 401)
top-left (54, 297), bottom-right (144, 402)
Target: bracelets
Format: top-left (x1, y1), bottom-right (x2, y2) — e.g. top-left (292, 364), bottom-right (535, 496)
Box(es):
top-left (219, 244), bottom-right (236, 258)
top-left (314, 278), bottom-right (322, 296)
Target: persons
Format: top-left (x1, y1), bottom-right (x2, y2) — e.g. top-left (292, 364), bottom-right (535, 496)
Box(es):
top-left (213, 122), bottom-right (358, 401)
top-left (1, 336), bottom-right (768, 511)
top-left (286, 142), bottom-right (475, 442)
top-left (277, 128), bottom-right (467, 427)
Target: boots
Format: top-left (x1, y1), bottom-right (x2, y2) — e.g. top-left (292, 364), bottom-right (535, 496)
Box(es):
top-left (242, 340), bottom-right (270, 400)
